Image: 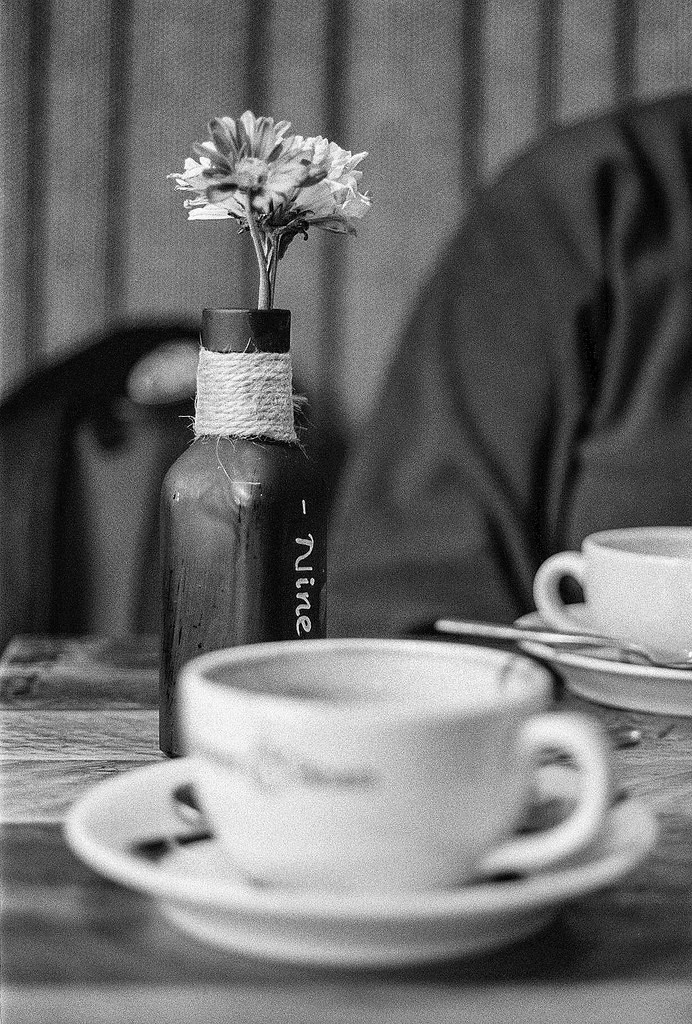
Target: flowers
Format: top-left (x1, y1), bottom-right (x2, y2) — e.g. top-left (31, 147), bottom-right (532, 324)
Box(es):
top-left (167, 109), bottom-right (374, 306)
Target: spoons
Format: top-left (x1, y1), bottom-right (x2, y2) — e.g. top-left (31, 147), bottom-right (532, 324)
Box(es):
top-left (434, 617), bottom-right (692, 669)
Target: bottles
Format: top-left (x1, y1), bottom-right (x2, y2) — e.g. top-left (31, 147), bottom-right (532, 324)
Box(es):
top-left (158, 309), bottom-right (327, 761)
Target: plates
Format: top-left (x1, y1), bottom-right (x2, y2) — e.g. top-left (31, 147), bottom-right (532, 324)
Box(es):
top-left (62, 756), bottom-right (660, 971)
top-left (512, 601), bottom-right (692, 720)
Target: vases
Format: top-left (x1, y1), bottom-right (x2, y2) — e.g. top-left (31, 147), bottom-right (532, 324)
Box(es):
top-left (159, 309), bottom-right (328, 757)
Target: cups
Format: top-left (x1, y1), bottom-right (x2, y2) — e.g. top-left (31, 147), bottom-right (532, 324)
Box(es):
top-left (533, 525), bottom-right (692, 668)
top-left (175, 638), bottom-right (616, 887)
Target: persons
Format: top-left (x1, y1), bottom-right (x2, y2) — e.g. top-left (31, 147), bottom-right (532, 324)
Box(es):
top-left (314, 89), bottom-right (692, 645)
